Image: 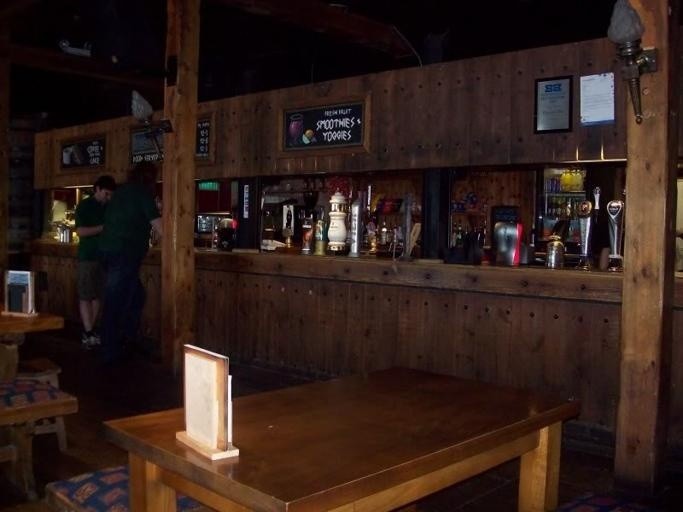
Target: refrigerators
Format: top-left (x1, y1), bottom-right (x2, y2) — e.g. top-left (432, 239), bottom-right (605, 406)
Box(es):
top-left (533, 164), bottom-right (617, 255)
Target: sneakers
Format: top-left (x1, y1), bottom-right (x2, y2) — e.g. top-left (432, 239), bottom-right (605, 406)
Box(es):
top-left (81, 334), bottom-right (101, 350)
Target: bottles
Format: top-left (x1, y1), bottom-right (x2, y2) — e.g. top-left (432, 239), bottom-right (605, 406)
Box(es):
top-left (545, 169), bottom-right (587, 220)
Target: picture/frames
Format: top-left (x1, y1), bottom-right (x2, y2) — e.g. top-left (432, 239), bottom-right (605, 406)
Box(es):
top-left (534, 75), bottom-right (573, 135)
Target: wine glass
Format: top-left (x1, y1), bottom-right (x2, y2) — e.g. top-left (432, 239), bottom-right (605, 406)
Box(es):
top-left (287, 114), bottom-right (305, 146)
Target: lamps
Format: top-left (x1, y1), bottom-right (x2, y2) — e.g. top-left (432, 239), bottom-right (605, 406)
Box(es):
top-left (607, 0), bottom-right (657, 124)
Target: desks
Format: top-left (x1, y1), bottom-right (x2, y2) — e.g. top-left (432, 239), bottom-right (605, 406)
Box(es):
top-left (0, 303), bottom-right (65, 379)
top-left (102, 366), bottom-right (581, 512)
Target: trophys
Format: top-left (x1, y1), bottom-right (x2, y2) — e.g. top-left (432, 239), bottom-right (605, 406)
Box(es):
top-left (604, 198), bottom-right (623, 272)
top-left (572, 199), bottom-right (595, 271)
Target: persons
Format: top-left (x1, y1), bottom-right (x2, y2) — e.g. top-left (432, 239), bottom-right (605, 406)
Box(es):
top-left (98, 160), bottom-right (162, 369)
top-left (74, 175), bottom-right (116, 350)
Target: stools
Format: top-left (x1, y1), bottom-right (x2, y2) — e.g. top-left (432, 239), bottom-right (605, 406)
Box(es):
top-left (45, 466), bottom-right (209, 512)
top-left (0, 379), bottom-right (79, 504)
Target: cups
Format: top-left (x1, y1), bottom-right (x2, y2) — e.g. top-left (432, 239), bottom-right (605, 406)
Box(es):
top-left (62, 147), bottom-right (74, 165)
